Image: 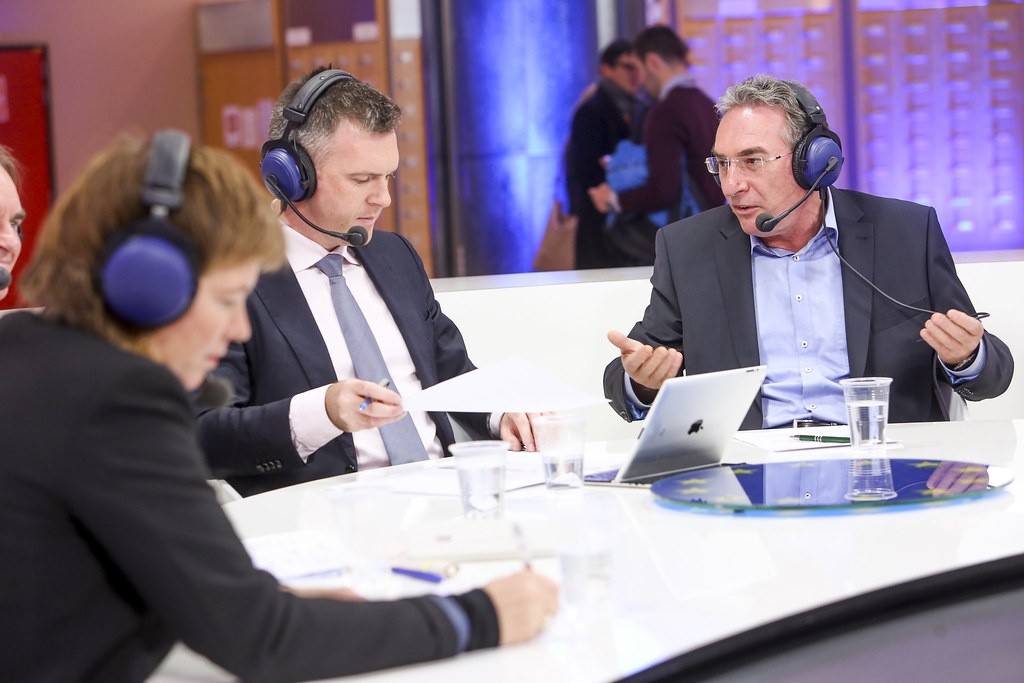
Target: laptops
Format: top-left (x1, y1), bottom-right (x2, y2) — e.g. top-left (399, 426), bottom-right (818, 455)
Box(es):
top-left (583, 363), bottom-right (769, 485)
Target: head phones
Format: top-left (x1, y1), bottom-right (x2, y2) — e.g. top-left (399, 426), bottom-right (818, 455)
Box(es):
top-left (88, 132), bottom-right (200, 336)
top-left (781, 80), bottom-right (844, 194)
top-left (260, 69), bottom-right (356, 212)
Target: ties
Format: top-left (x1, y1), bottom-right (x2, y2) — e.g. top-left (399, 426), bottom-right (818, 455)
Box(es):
top-left (315, 253), bottom-right (429, 466)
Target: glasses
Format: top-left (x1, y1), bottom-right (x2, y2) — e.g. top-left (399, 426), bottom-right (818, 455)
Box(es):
top-left (621, 62), bottom-right (637, 73)
top-left (705, 152), bottom-right (794, 175)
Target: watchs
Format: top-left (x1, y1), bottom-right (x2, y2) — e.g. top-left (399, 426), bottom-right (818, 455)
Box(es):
top-left (954, 353), bottom-right (976, 371)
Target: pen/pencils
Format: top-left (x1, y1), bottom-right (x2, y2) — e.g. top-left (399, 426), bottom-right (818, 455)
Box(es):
top-left (790, 435), bottom-right (850, 443)
top-left (526, 562), bottom-right (533, 572)
top-left (359, 378), bottom-right (390, 412)
top-left (392, 568), bottom-right (442, 583)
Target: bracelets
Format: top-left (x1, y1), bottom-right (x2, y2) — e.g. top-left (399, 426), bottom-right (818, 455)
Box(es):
top-left (608, 192), bottom-right (621, 214)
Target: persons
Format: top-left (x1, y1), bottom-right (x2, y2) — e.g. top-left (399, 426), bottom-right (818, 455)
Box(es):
top-left (0, 146), bottom-right (26, 300)
top-left (603, 73), bottom-right (1015, 431)
top-left (589, 25), bottom-right (727, 269)
top-left (0, 131), bottom-right (559, 683)
top-left (566, 39), bottom-right (648, 271)
top-left (196, 65), bottom-right (557, 498)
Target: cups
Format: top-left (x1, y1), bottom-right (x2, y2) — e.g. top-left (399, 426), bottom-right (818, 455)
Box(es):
top-left (449, 440), bottom-right (510, 521)
top-left (841, 376), bottom-right (892, 447)
top-left (844, 459), bottom-right (896, 500)
top-left (531, 415), bottom-right (584, 486)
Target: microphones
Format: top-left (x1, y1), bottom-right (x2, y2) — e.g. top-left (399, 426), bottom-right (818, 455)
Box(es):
top-left (275, 185), bottom-right (369, 246)
top-left (0, 266), bottom-right (11, 290)
top-left (755, 171), bottom-right (825, 231)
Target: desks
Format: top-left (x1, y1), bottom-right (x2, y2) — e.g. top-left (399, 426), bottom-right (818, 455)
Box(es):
top-left (142, 421), bottom-right (1023, 683)
